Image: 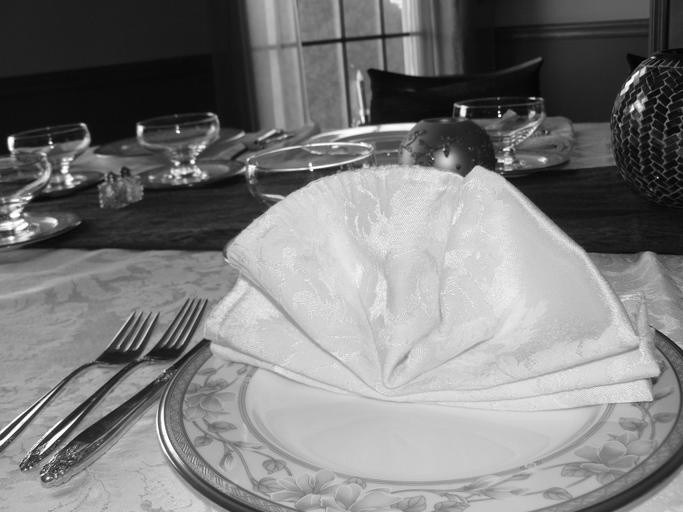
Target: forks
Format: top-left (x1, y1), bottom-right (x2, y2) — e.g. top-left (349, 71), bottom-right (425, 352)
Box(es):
top-left (0, 296), bottom-right (208, 471)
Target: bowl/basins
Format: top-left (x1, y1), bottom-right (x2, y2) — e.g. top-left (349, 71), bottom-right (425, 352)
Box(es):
top-left (245, 139), bottom-right (373, 209)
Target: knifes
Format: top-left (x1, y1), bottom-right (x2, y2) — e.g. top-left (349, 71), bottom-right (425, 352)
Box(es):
top-left (38, 338), bottom-right (211, 488)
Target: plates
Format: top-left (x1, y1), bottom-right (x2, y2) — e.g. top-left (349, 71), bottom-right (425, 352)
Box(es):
top-left (133, 158), bottom-right (247, 190)
top-left (494, 150), bottom-right (572, 175)
top-left (0, 210), bottom-right (83, 252)
top-left (94, 129), bottom-right (245, 161)
top-left (29, 170), bottom-right (103, 201)
top-left (158, 317), bottom-right (682, 512)
top-left (332, 131), bottom-right (410, 166)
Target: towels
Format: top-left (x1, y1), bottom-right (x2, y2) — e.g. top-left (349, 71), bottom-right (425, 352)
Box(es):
top-left (203, 162), bottom-right (661, 408)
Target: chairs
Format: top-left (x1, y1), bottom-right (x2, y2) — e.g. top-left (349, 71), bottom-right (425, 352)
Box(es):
top-left (367, 57), bottom-right (544, 124)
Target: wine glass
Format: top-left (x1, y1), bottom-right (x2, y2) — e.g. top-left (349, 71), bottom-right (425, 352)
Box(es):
top-left (7, 122), bottom-right (92, 189)
top-left (136, 112), bottom-right (220, 182)
top-left (0, 152), bottom-right (53, 242)
top-left (452, 97), bottom-right (547, 172)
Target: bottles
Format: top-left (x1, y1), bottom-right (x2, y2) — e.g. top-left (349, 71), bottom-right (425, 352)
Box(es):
top-left (98, 166), bottom-right (144, 210)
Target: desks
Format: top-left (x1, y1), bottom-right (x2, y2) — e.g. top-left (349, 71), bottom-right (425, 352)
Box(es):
top-left (0, 119), bottom-right (683, 512)
